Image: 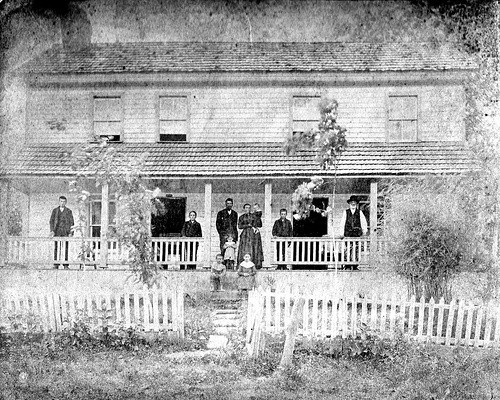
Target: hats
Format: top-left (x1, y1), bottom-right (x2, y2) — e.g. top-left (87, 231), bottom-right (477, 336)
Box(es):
top-left (347, 195), bottom-right (359, 204)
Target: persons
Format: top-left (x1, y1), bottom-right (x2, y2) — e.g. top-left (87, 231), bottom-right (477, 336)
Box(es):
top-left (209, 254), bottom-right (226, 291)
top-left (340, 195), bottom-right (367, 271)
top-left (181, 210), bottom-right (202, 269)
top-left (236, 204), bottom-right (263, 269)
top-left (216, 198), bottom-right (238, 264)
top-left (272, 208), bottom-right (293, 270)
top-left (50, 196), bottom-right (73, 269)
top-left (252, 203), bottom-right (263, 235)
top-left (223, 234), bottom-right (236, 269)
top-left (238, 253), bottom-right (257, 293)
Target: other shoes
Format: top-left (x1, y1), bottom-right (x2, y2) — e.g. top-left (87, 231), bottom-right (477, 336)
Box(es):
top-left (64, 266), bottom-right (69, 270)
top-left (52, 266), bottom-right (58, 269)
